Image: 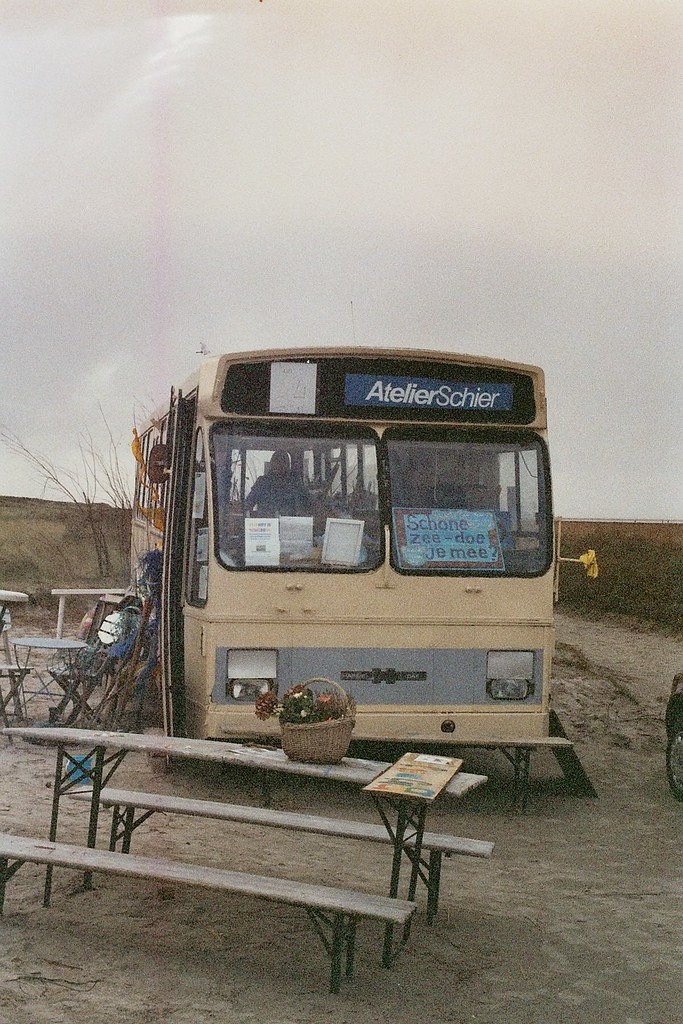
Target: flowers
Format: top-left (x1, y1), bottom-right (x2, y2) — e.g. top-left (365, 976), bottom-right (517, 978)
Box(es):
top-left (256, 685), bottom-right (346, 724)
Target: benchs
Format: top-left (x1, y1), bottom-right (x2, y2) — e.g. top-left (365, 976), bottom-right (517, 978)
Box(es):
top-left (69, 786), bottom-right (495, 925)
top-left (0, 833), bottom-right (418, 994)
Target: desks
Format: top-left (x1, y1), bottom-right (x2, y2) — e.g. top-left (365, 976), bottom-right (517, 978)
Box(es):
top-left (0, 728), bottom-right (489, 965)
top-left (9, 638), bottom-right (88, 727)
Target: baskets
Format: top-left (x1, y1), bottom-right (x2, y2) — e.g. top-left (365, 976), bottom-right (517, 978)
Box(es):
top-left (278, 678), bottom-right (356, 765)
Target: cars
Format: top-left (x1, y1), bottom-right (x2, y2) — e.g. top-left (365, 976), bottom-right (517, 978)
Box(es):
top-left (664, 673), bottom-right (683, 798)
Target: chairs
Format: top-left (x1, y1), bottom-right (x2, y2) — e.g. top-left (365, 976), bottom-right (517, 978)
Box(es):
top-left (0, 607), bottom-right (34, 728)
top-left (50, 613), bottom-right (123, 727)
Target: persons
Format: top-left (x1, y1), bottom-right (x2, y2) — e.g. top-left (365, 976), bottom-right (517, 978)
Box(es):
top-left (230, 450), bottom-right (312, 518)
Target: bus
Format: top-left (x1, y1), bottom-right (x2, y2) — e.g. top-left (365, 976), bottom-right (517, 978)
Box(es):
top-left (130, 349), bottom-right (601, 753)
